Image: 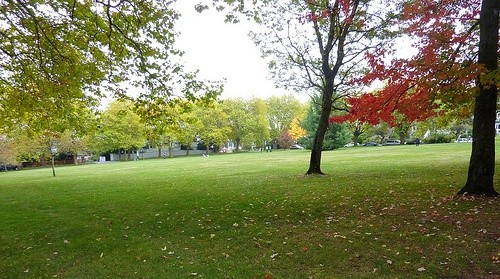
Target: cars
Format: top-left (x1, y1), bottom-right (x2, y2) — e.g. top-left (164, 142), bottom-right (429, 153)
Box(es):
top-left (344, 142), bottom-right (360, 147)
top-left (406, 140), bottom-right (424, 145)
top-left (365, 142), bottom-right (378, 147)
top-left (290, 145), bottom-right (303, 150)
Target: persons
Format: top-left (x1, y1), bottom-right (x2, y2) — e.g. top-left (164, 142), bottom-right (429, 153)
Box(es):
top-left (269, 145), bottom-right (271, 152)
top-left (203, 153), bottom-right (209, 158)
top-left (136, 150), bottom-right (140, 161)
top-left (163, 153), bottom-right (166, 159)
top-left (456, 135), bottom-right (461, 143)
top-left (266, 145), bottom-right (268, 152)
top-left (466, 135), bottom-right (471, 143)
top-left (414, 137), bottom-right (419, 146)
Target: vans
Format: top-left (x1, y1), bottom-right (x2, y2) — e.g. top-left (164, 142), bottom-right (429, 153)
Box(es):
top-left (385, 140), bottom-right (401, 144)
